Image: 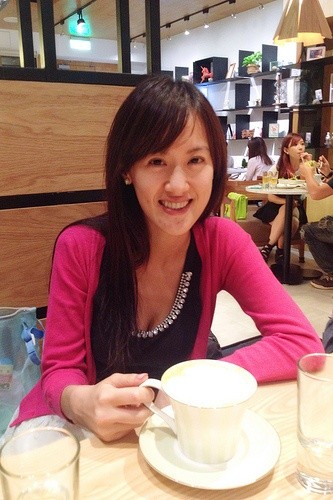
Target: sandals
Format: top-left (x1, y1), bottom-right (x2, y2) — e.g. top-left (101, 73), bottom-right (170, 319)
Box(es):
top-left (260, 242), bottom-right (275, 262)
top-left (275, 246), bottom-right (284, 266)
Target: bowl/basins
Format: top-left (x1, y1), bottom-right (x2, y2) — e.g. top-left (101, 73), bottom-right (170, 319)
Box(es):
top-left (277, 179), bottom-right (305, 186)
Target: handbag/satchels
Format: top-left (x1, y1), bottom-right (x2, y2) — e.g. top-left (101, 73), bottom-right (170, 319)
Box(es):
top-left (0, 306), bottom-right (48, 436)
top-left (225, 192), bottom-right (248, 220)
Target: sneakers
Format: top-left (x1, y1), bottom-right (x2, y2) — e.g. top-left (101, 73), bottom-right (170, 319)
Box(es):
top-left (310, 273), bottom-right (333, 290)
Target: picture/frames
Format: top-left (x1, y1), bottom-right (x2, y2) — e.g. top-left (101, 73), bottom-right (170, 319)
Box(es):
top-left (306, 46), bottom-right (326, 61)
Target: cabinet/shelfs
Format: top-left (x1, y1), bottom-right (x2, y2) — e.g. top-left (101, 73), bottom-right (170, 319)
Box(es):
top-left (161, 43), bottom-right (333, 140)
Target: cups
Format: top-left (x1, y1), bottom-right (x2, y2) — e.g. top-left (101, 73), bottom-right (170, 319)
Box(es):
top-left (263, 170), bottom-right (279, 189)
top-left (314, 174), bottom-right (325, 186)
top-left (0, 426), bottom-right (81, 500)
top-left (138, 359), bottom-right (258, 465)
top-left (295, 353), bottom-right (333, 495)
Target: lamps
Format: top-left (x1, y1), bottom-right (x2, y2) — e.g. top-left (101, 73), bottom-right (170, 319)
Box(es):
top-left (273, 0), bottom-right (333, 46)
top-left (76, 9), bottom-right (86, 34)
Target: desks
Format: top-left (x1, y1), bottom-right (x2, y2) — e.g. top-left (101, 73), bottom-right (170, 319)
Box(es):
top-left (227, 167), bottom-right (247, 179)
top-left (245, 184), bottom-right (309, 283)
top-left (0, 378), bottom-right (333, 499)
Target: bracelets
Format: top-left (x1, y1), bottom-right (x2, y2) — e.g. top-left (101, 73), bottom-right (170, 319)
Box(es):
top-left (325, 168), bottom-right (333, 179)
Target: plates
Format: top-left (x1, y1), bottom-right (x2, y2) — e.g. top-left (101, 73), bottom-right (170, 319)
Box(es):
top-left (139, 405), bottom-right (282, 490)
top-left (277, 185), bottom-right (298, 188)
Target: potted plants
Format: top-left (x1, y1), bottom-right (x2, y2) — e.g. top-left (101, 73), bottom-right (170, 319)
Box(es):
top-left (241, 51), bottom-right (262, 74)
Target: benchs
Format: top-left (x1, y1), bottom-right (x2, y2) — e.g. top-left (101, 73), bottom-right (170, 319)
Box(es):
top-left (222, 179), bottom-right (307, 263)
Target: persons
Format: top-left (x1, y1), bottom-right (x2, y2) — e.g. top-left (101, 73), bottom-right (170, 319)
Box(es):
top-left (252, 133), bottom-right (315, 268)
top-left (238, 137), bottom-right (274, 205)
top-left (297, 155), bottom-right (333, 290)
top-left (0, 74), bottom-right (327, 446)
